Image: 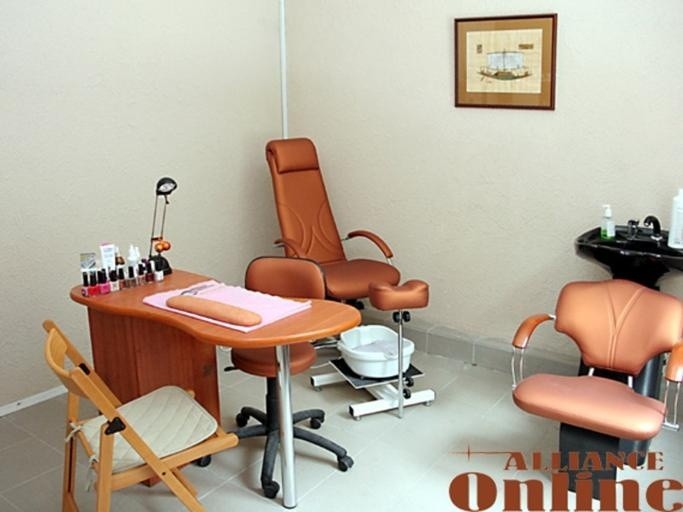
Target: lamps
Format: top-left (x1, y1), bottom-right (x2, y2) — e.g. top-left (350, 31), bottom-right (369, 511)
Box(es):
top-left (147, 177), bottom-right (178, 276)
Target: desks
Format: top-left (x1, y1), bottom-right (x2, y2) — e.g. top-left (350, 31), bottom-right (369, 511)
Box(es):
top-left (69, 270), bottom-right (362, 509)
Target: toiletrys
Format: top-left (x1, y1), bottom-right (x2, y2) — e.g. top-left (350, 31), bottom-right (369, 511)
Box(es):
top-left (81, 241), bottom-right (164, 297)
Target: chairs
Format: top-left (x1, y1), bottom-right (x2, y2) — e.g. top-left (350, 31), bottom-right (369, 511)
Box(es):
top-left (43, 320), bottom-right (240, 512)
top-left (265, 137), bottom-right (401, 303)
top-left (197, 255), bottom-right (354, 499)
top-left (510, 279), bottom-right (683, 500)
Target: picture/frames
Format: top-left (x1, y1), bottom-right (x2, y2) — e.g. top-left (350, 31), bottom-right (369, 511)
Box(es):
top-left (454, 13), bottom-right (557, 111)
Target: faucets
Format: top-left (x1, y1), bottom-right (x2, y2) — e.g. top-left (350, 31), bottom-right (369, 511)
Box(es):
top-left (644, 216), bottom-right (663, 241)
